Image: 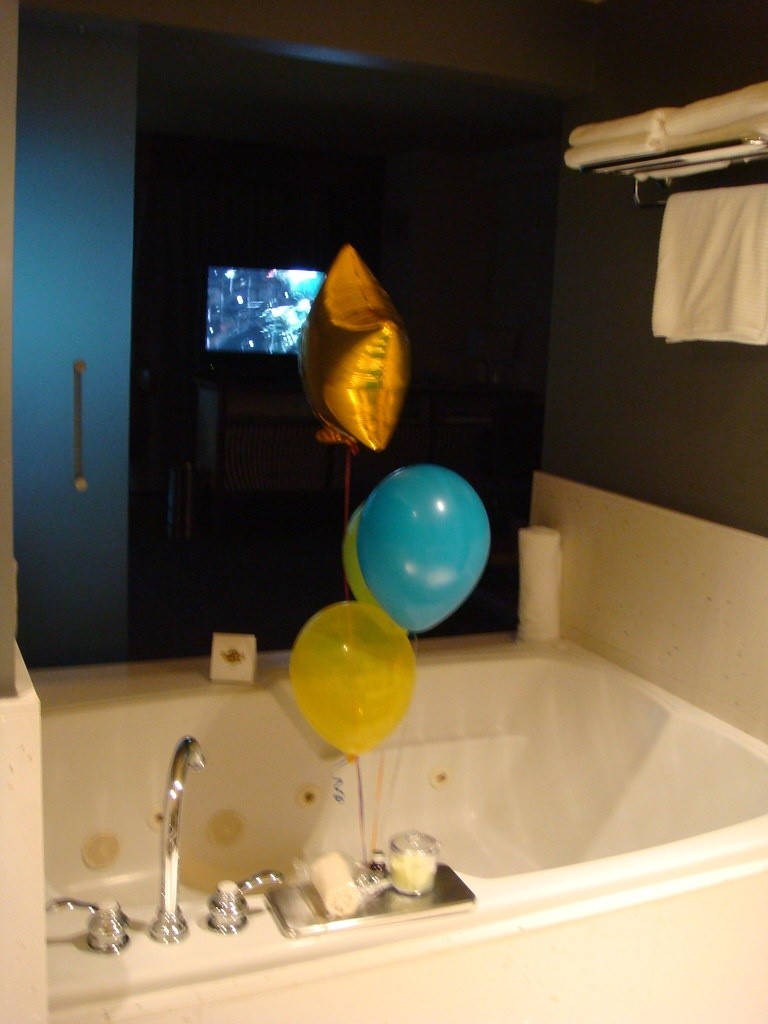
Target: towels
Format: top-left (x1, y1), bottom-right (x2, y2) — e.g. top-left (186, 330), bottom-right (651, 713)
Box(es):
top-left (563, 80), bottom-right (768, 168)
top-left (651, 183), bottom-right (767, 346)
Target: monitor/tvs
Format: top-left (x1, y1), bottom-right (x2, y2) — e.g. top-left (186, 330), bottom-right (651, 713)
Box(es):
top-left (206, 267), bottom-right (327, 355)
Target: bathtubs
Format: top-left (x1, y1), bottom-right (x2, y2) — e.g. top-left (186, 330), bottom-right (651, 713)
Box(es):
top-left (42, 631), bottom-right (766, 1024)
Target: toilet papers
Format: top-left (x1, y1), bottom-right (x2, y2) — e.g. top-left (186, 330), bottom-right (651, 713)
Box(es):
top-left (309, 850), bottom-right (362, 918)
top-left (516, 524), bottom-right (562, 644)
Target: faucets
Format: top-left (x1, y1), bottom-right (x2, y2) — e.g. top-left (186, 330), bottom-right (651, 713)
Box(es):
top-left (147, 735), bottom-right (208, 945)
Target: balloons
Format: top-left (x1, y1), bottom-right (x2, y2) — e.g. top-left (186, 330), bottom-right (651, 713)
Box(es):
top-left (355, 464), bottom-right (491, 634)
top-left (288, 602), bottom-right (418, 760)
top-left (344, 507), bottom-right (412, 637)
top-left (299, 241), bottom-right (410, 452)
